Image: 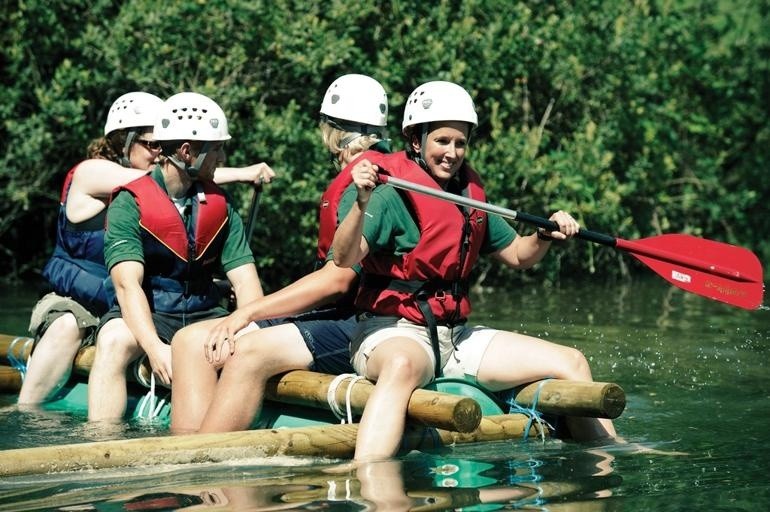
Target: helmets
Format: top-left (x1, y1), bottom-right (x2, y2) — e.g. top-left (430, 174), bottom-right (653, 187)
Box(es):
top-left (102, 92), bottom-right (164, 136)
top-left (402, 80), bottom-right (479, 136)
top-left (153, 91), bottom-right (233, 142)
top-left (318, 73), bottom-right (389, 128)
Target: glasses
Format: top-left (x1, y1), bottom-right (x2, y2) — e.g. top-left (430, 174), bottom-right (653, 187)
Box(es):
top-left (132, 139), bottom-right (160, 150)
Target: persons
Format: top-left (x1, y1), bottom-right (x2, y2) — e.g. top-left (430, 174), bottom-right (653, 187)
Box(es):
top-left (167, 73), bottom-right (396, 434)
top-left (330, 80), bottom-right (621, 460)
top-left (17, 91), bottom-right (276, 405)
top-left (86, 90), bottom-right (264, 422)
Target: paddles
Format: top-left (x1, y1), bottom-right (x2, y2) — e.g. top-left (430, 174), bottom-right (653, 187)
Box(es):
top-left (375, 172), bottom-right (763, 310)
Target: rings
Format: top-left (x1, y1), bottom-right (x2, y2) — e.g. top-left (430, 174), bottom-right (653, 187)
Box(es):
top-left (258, 175), bottom-right (263, 180)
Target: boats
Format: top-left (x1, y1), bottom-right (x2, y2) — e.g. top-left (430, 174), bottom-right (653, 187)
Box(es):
top-left (0, 312), bottom-right (628, 477)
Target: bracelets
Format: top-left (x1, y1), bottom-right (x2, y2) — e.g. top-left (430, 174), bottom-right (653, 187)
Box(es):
top-left (537, 230), bottom-right (554, 242)
